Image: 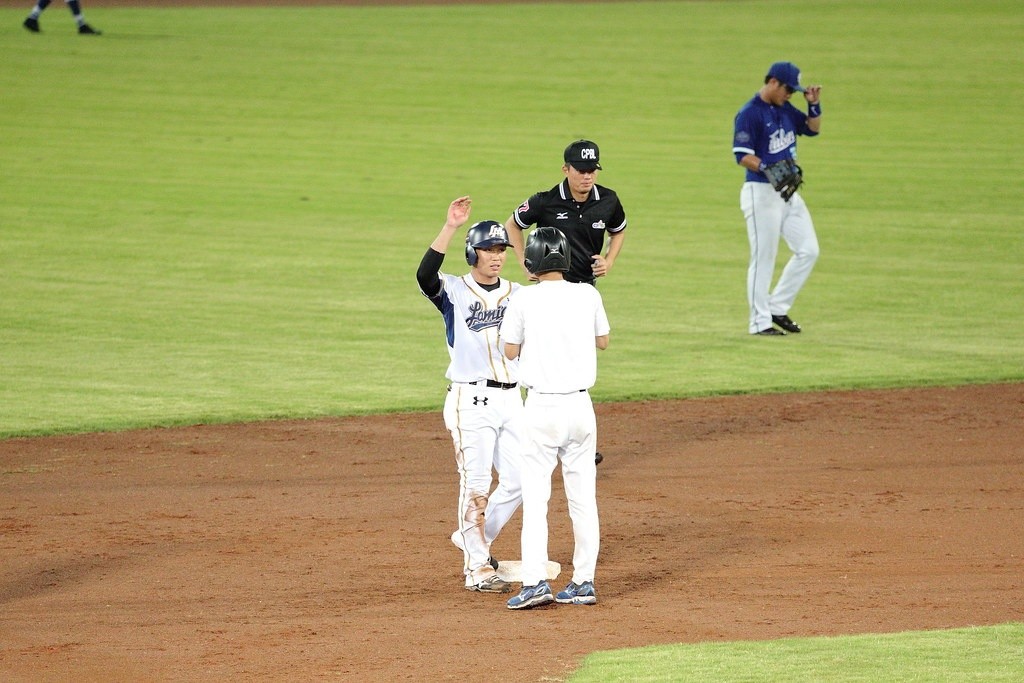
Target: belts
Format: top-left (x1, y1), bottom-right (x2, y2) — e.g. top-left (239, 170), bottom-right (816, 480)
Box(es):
top-left (540, 389), bottom-right (586, 394)
top-left (469, 379), bottom-right (517, 390)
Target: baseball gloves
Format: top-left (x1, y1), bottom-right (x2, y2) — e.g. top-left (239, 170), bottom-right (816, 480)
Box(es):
top-left (763, 159), bottom-right (803, 202)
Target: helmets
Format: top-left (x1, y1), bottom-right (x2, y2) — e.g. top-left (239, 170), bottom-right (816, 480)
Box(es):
top-left (524, 227), bottom-right (571, 274)
top-left (465, 220), bottom-right (514, 266)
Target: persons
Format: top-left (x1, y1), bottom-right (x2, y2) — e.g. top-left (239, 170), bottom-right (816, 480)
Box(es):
top-left (24, 0), bottom-right (100, 35)
top-left (505, 139), bottom-right (626, 465)
top-left (501, 226), bottom-right (610, 612)
top-left (732, 62), bottom-right (822, 336)
top-left (417, 195), bottom-right (524, 592)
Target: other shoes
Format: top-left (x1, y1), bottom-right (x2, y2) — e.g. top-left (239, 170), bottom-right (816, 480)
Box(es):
top-left (772, 315), bottom-right (801, 332)
top-left (755, 328), bottom-right (787, 335)
top-left (24, 18), bottom-right (39, 32)
top-left (80, 24), bottom-right (100, 35)
top-left (595, 452), bottom-right (603, 466)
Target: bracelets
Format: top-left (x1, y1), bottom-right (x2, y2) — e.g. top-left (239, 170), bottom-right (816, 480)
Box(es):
top-left (758, 161), bottom-right (768, 172)
top-left (808, 101), bottom-right (821, 116)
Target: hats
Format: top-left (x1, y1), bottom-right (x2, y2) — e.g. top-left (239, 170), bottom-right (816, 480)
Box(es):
top-left (564, 139), bottom-right (602, 171)
top-left (769, 62), bottom-right (807, 93)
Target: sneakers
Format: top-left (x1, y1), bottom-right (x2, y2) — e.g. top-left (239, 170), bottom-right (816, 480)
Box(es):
top-left (465, 575), bottom-right (513, 593)
top-left (506, 580), bottom-right (554, 609)
top-left (451, 530), bottom-right (499, 570)
top-left (556, 581), bottom-right (596, 604)
